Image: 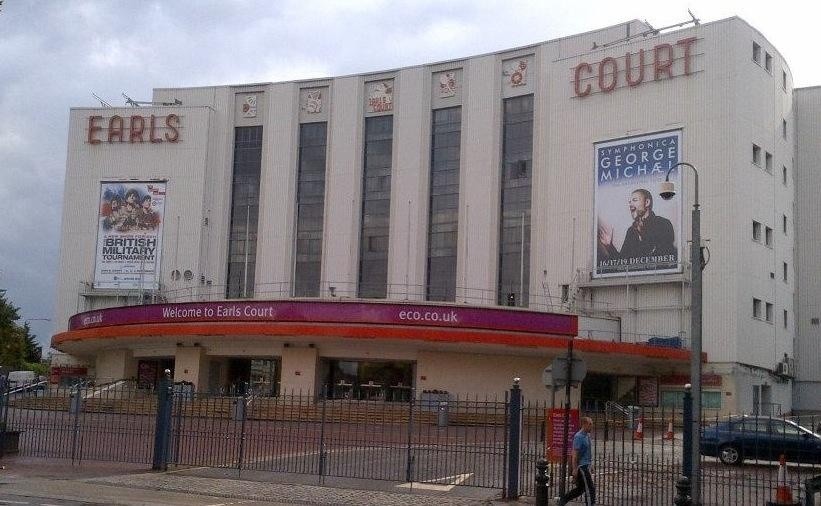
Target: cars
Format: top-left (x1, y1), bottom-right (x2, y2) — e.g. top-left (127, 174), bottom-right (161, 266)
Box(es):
top-left (700, 415), bottom-right (821, 467)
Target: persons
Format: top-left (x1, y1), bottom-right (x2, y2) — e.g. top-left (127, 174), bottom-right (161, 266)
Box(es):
top-left (597, 188), bottom-right (676, 265)
top-left (552, 416), bottom-right (596, 506)
top-left (102, 189), bottom-right (160, 232)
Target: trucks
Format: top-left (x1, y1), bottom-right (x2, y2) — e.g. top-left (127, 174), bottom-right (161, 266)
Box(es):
top-left (7, 371), bottom-right (34, 387)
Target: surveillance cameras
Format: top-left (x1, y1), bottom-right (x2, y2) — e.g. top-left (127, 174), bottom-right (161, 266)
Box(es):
top-left (659, 181), bottom-right (676, 200)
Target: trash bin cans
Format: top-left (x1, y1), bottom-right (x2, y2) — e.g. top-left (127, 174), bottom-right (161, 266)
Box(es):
top-left (628, 406), bottom-right (639, 429)
top-left (420, 390), bottom-right (448, 412)
top-left (438, 402), bottom-right (448, 426)
top-left (173, 382), bottom-right (193, 401)
top-left (69, 391), bottom-right (79, 414)
top-left (233, 396), bottom-right (246, 419)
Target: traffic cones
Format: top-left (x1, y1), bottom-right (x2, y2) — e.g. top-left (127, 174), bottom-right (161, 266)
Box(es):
top-left (633, 415), bottom-right (644, 440)
top-left (765, 454), bottom-right (801, 504)
top-left (665, 412), bottom-right (676, 440)
